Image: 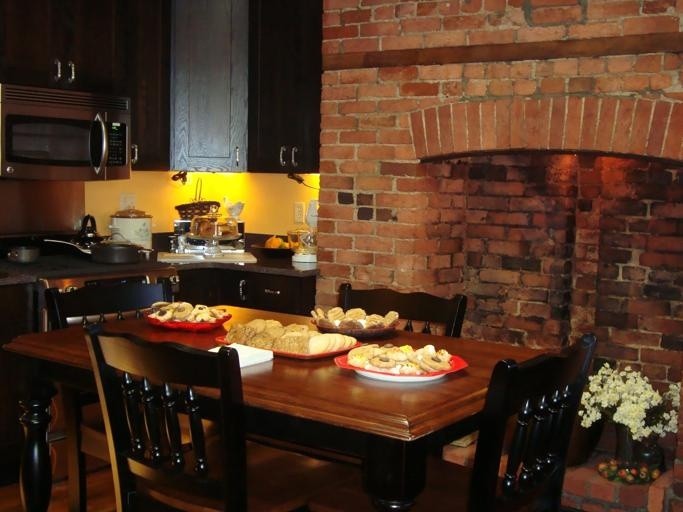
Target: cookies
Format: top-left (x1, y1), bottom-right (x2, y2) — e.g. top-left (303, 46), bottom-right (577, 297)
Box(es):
top-left (224, 319), bottom-right (357, 356)
top-left (151, 301), bottom-right (226, 323)
top-left (310, 306), bottom-right (399, 328)
top-left (348, 343), bottom-right (451, 375)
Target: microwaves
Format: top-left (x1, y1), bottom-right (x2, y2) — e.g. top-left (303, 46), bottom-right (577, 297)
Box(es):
top-left (0, 82), bottom-right (131, 182)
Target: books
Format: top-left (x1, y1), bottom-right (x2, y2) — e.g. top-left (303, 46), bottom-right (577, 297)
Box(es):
top-left (207, 343), bottom-right (273, 368)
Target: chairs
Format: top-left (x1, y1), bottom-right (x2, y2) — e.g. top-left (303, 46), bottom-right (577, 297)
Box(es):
top-left (242, 280), bottom-right (468, 468)
top-left (76, 319), bottom-right (358, 512)
top-left (299, 329), bottom-right (602, 512)
top-left (39, 273), bottom-right (224, 512)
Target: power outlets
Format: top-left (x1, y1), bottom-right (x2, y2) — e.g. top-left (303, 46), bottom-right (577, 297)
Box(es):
top-left (292, 201), bottom-right (304, 225)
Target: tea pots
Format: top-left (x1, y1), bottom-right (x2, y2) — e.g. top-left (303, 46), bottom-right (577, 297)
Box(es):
top-left (77, 215), bottom-right (97, 238)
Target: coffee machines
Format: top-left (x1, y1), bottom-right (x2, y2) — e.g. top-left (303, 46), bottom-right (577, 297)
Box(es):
top-left (291, 200), bottom-right (318, 263)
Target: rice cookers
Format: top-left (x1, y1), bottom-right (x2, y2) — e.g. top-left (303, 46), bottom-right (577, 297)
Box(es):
top-left (107, 206), bottom-right (153, 249)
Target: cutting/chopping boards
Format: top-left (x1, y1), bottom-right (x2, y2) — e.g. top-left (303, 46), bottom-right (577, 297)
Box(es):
top-left (155, 250), bottom-right (258, 265)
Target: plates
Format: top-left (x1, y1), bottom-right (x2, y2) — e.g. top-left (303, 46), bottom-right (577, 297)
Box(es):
top-left (140, 309), bottom-right (232, 332)
top-left (309, 320), bottom-right (401, 338)
top-left (250, 244), bottom-right (296, 251)
top-left (333, 350), bottom-right (468, 384)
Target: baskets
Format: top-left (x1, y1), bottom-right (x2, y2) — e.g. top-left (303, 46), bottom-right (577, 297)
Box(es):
top-left (174, 178), bottom-right (220, 220)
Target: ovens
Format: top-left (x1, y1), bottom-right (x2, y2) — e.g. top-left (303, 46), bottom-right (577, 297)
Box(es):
top-left (38, 271), bottom-right (180, 331)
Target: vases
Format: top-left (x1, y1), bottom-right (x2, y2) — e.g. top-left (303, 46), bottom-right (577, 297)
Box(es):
top-left (609, 416), bottom-right (636, 465)
top-left (635, 436), bottom-right (665, 473)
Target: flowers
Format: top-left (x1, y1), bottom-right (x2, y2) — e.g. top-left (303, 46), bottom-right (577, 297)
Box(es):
top-left (579, 361), bottom-right (682, 456)
top-left (598, 455), bottom-right (660, 486)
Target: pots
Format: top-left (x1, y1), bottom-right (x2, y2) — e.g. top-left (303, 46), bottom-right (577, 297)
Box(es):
top-left (43, 238), bottom-right (143, 265)
top-left (5, 246), bottom-right (41, 264)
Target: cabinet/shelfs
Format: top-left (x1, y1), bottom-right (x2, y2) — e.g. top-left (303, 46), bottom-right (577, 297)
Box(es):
top-left (215, 265), bottom-right (316, 315)
top-left (160, 265), bottom-right (216, 307)
top-left (115, 0), bottom-right (170, 172)
top-left (1, 0), bottom-right (114, 94)
top-left (0, 281), bottom-right (47, 485)
top-left (246, 0), bottom-right (321, 174)
top-left (170, 0), bottom-right (249, 174)
